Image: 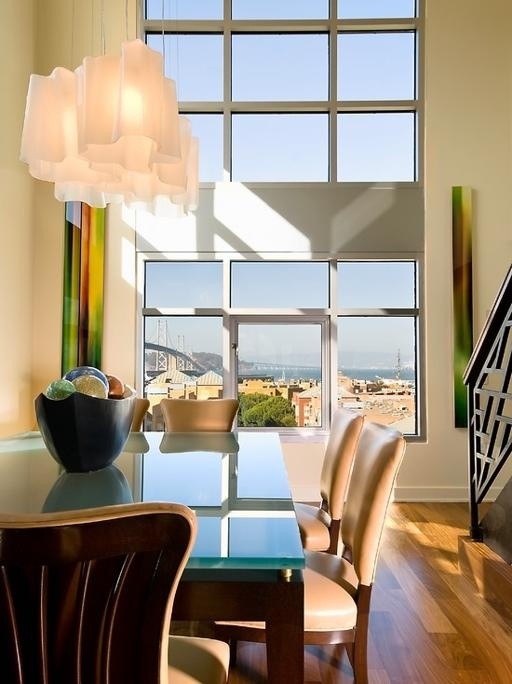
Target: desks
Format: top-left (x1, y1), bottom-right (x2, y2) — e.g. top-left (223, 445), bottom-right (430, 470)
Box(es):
top-left (0, 429), bottom-right (308, 684)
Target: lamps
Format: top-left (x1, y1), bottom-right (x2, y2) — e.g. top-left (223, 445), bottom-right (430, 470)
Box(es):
top-left (20, 0), bottom-right (201, 219)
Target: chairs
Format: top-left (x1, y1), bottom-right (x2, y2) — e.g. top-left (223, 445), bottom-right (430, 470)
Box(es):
top-left (0, 500), bottom-right (235, 684)
top-left (292, 405), bottom-right (368, 554)
top-left (153, 397), bottom-right (241, 433)
top-left (128, 399), bottom-right (151, 432)
top-left (210, 420), bottom-right (407, 684)
